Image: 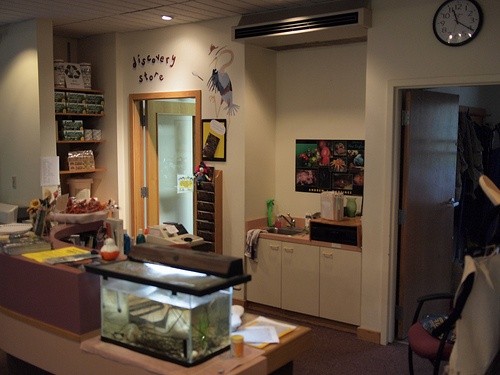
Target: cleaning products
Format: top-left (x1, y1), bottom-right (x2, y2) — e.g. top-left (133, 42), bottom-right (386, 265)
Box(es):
top-left (266, 198), bottom-right (275, 227)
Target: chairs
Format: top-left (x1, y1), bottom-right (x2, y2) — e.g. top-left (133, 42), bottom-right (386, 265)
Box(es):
top-left (407, 293), bottom-right (463, 374)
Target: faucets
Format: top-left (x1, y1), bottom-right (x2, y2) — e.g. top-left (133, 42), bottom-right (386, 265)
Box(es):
top-left (276, 212), bottom-right (296, 228)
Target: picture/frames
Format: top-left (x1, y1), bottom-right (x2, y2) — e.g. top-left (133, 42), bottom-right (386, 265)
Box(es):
top-left (201, 118), bottom-right (227, 162)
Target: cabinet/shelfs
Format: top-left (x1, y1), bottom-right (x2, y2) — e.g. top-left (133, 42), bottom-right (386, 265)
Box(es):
top-left (53, 86), bottom-right (105, 174)
top-left (245, 237), bottom-right (361, 327)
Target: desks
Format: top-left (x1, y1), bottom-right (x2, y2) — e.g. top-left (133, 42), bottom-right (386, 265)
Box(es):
top-left (0, 306), bottom-right (313, 375)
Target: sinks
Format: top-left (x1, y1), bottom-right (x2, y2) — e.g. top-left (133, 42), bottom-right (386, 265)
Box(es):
top-left (263, 228), bottom-right (302, 236)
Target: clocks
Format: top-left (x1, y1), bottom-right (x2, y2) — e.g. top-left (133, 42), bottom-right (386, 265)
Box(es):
top-left (433, 0), bottom-right (483, 47)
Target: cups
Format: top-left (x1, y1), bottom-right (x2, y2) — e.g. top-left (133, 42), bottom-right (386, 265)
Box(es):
top-left (231, 335), bottom-right (244, 358)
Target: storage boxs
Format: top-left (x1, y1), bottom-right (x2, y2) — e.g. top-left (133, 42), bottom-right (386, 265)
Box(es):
top-left (85, 243), bottom-right (252, 369)
top-left (54, 91), bottom-right (104, 172)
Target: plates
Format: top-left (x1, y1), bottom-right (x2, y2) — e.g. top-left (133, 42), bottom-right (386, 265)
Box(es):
top-left (0, 223), bottom-right (34, 236)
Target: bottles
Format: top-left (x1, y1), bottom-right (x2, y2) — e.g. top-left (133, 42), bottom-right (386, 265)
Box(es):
top-left (136, 229), bottom-right (145, 244)
top-left (346, 197), bottom-right (357, 217)
